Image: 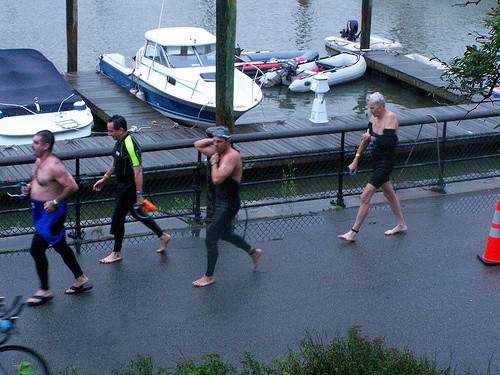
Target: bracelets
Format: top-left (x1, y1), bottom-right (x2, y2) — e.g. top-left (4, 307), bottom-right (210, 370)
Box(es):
top-left (103, 174), bottom-right (111, 179)
top-left (355, 153), bottom-right (360, 157)
top-left (136, 190), bottom-right (144, 195)
top-left (367, 136), bottom-right (371, 141)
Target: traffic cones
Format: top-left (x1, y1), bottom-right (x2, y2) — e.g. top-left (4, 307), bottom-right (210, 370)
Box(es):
top-left (476, 200), bottom-right (500, 266)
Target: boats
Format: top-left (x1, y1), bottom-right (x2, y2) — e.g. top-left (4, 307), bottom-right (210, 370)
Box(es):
top-left (0, 48), bottom-right (94, 145)
top-left (234, 43), bottom-right (319, 76)
top-left (259, 50), bottom-right (368, 93)
top-left (96, 26), bottom-right (265, 126)
top-left (324, 29), bottom-right (403, 55)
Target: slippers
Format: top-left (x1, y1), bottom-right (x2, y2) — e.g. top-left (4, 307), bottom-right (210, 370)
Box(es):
top-left (65, 285), bottom-right (93, 294)
top-left (26, 295), bottom-right (53, 306)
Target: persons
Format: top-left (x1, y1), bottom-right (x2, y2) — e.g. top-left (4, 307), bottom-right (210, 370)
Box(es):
top-left (20, 130), bottom-right (92, 306)
top-left (337, 91), bottom-right (407, 242)
top-left (92, 114), bottom-right (173, 263)
top-left (191, 125), bottom-right (262, 287)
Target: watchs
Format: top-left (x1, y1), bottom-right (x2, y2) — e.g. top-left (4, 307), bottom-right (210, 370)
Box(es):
top-left (210, 162), bottom-right (219, 166)
top-left (53, 199), bottom-right (59, 207)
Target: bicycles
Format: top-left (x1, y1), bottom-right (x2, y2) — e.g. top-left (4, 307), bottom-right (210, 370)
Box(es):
top-left (0, 294), bottom-right (52, 375)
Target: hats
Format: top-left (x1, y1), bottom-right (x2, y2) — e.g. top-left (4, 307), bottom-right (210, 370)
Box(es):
top-left (206, 126), bottom-right (230, 138)
